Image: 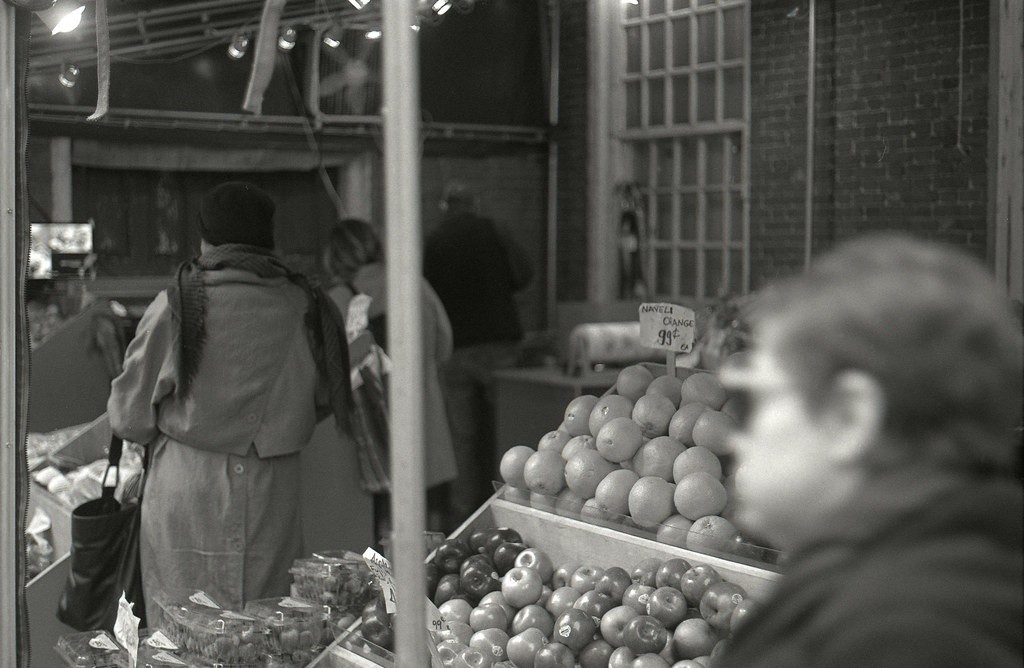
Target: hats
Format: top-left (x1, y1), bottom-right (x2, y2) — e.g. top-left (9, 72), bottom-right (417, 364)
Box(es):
top-left (196, 181), bottom-right (274, 251)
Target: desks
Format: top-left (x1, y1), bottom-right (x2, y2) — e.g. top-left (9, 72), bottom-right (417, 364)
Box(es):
top-left (491, 364), bottom-right (630, 493)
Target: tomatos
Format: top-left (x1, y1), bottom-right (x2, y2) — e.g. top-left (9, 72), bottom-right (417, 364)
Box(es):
top-left (362, 526), bottom-right (760, 668)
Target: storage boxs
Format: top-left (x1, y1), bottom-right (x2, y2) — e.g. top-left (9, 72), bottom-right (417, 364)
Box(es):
top-left (303, 360), bottom-right (790, 668)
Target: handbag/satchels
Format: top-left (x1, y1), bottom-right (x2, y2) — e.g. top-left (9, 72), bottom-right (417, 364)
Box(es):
top-left (57, 497), bottom-right (148, 629)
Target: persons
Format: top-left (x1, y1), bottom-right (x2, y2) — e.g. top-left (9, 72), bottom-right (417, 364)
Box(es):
top-left (710, 232), bottom-right (1024, 668)
top-left (108, 186), bottom-right (352, 631)
top-left (322, 188), bottom-right (534, 555)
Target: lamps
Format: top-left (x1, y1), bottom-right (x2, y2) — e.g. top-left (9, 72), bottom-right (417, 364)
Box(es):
top-left (32, 3), bottom-right (87, 88)
top-left (225, 16), bottom-right (345, 62)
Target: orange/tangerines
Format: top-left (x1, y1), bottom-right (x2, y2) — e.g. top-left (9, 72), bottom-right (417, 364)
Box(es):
top-left (499, 366), bottom-right (761, 552)
